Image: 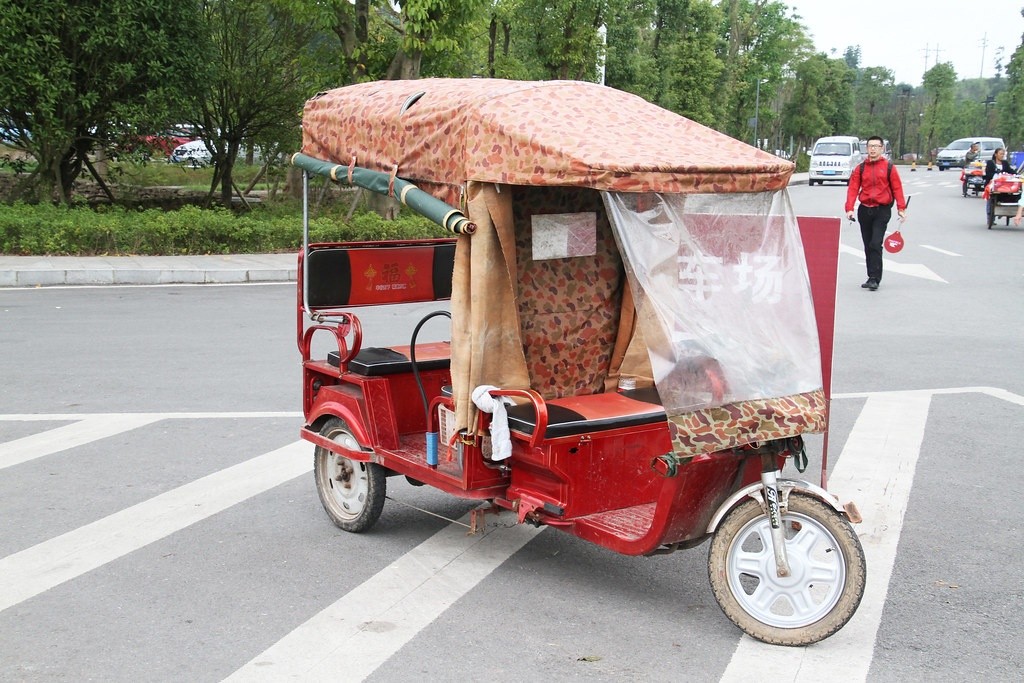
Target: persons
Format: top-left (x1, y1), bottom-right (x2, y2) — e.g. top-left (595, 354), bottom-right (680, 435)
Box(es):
top-left (845, 136), bottom-right (906, 290)
top-left (961, 144), bottom-right (980, 196)
top-left (984, 148), bottom-right (1020, 226)
top-left (1012, 191), bottom-right (1024, 226)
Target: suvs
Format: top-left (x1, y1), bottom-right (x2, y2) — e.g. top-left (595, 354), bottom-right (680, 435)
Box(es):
top-left (859, 139), bottom-right (892, 162)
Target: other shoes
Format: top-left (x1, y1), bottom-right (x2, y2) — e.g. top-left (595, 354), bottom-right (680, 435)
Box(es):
top-left (862, 278), bottom-right (871, 288)
top-left (868, 277), bottom-right (879, 289)
top-left (962, 193), bottom-right (970, 197)
top-left (987, 221), bottom-right (996, 225)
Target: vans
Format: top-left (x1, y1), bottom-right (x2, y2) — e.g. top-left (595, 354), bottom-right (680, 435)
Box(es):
top-left (936, 137), bottom-right (1008, 171)
top-left (807, 136), bottom-right (861, 186)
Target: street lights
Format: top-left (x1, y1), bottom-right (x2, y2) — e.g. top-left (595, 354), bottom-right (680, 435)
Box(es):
top-left (980, 95), bottom-right (997, 137)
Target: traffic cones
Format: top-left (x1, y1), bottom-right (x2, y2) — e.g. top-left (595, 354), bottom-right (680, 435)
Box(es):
top-left (910, 160), bottom-right (916, 171)
top-left (928, 162), bottom-right (933, 170)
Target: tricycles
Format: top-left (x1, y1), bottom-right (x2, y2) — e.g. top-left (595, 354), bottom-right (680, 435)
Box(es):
top-left (988, 169), bottom-right (1024, 228)
top-left (291, 77), bottom-right (866, 645)
top-left (963, 160), bottom-right (986, 197)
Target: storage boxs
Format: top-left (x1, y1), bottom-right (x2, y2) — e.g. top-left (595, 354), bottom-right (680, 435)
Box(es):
top-left (438, 403), bottom-right (458, 450)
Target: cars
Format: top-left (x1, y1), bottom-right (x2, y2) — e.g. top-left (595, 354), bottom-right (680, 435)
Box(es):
top-left (119, 124), bottom-right (281, 168)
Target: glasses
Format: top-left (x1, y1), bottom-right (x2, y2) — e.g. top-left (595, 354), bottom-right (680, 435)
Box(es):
top-left (867, 144), bottom-right (883, 149)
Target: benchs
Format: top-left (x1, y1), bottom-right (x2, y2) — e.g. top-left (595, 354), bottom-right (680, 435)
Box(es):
top-left (297, 238), bottom-right (458, 376)
top-left (478, 364), bottom-right (725, 439)
